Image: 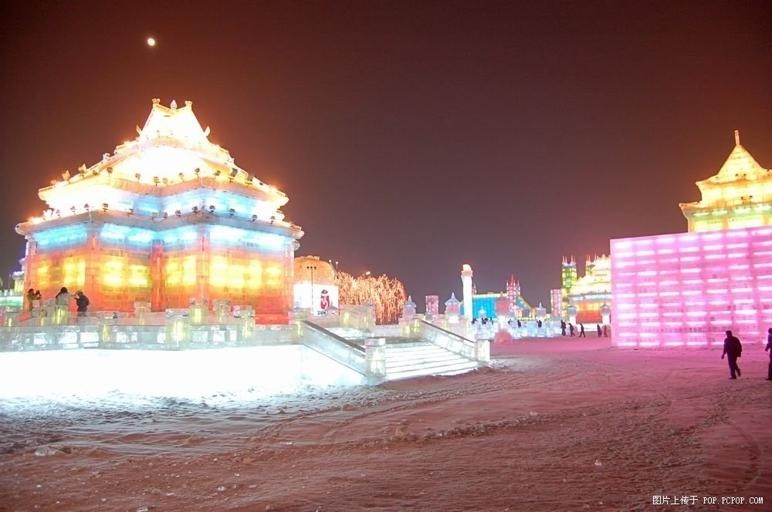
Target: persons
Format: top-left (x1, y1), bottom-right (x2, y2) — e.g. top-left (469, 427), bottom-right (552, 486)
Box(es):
top-left (569, 323), bottom-right (575, 337)
top-left (54, 287), bottom-right (74, 309)
top-left (604, 325), bottom-right (608, 337)
top-left (597, 324), bottom-right (602, 337)
top-left (721, 330), bottom-right (742, 379)
top-left (471, 316), bottom-right (493, 325)
top-left (764, 328), bottom-right (772, 381)
top-left (517, 320), bottom-right (521, 328)
top-left (508, 319), bottom-right (513, 325)
top-left (560, 320), bottom-right (566, 336)
top-left (579, 323), bottom-right (586, 338)
top-left (27, 288), bottom-right (40, 312)
top-left (35, 290), bottom-right (42, 300)
top-left (74, 289), bottom-right (89, 318)
top-left (538, 320), bottom-right (542, 328)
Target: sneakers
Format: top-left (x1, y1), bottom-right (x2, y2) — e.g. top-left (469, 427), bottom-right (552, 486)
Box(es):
top-left (728, 376), bottom-right (737, 380)
top-left (763, 377), bottom-right (772, 381)
top-left (737, 368), bottom-right (741, 377)
